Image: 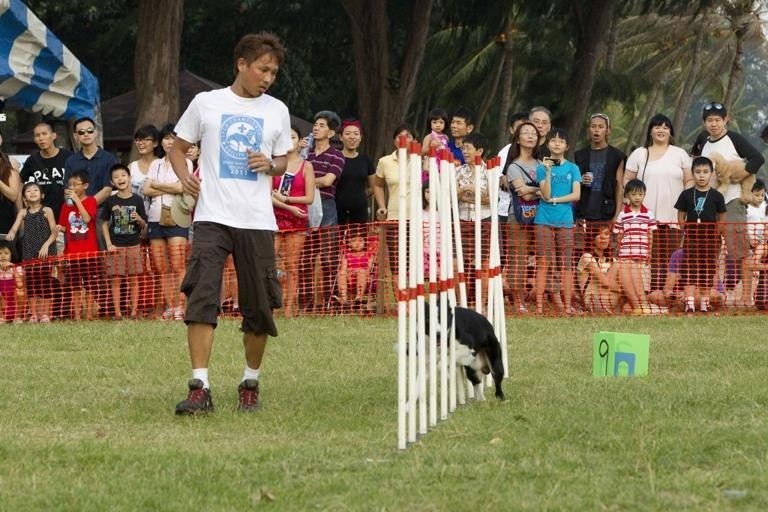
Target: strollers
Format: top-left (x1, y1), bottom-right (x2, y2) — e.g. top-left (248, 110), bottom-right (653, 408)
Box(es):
top-left (325, 230), bottom-right (379, 317)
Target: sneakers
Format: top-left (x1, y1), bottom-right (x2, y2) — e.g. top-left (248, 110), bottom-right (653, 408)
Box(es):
top-left (237, 379), bottom-right (260, 412)
top-left (175, 379), bottom-right (213, 415)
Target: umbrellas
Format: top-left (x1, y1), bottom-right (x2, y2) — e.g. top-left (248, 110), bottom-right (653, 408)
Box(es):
top-left (1, 1), bottom-right (101, 124)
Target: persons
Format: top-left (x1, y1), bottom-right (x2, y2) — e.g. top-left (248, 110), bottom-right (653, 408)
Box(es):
top-left (99, 162), bottom-right (150, 322)
top-left (142, 124), bottom-right (195, 323)
top-left (53, 169), bottom-right (98, 322)
top-left (4, 181), bottom-right (59, 324)
top-left (14, 121), bottom-right (76, 316)
top-left (168, 30), bottom-right (294, 418)
top-left (270, 109), bottom-right (491, 317)
top-left (0, 238), bottom-right (27, 326)
top-left (0, 125), bottom-right (21, 262)
top-left (125, 124), bottom-right (162, 274)
top-left (62, 116), bottom-right (121, 317)
top-left (498, 102), bottom-right (767, 318)
top-left (184, 141), bottom-right (201, 262)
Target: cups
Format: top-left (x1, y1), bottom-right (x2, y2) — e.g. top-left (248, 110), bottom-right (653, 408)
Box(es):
top-left (66, 198), bottom-right (74, 206)
top-left (587, 171), bottom-right (594, 183)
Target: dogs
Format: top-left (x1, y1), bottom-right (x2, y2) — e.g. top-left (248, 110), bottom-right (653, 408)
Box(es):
top-left (708, 153), bottom-right (756, 204)
top-left (406, 301), bottom-right (505, 401)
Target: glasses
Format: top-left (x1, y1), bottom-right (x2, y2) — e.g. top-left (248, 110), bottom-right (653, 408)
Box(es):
top-left (590, 113), bottom-right (609, 127)
top-left (134, 138), bottom-right (153, 144)
top-left (520, 131), bottom-right (537, 138)
top-left (76, 128), bottom-right (96, 135)
top-left (705, 103), bottom-right (724, 109)
top-left (65, 182), bottom-right (86, 187)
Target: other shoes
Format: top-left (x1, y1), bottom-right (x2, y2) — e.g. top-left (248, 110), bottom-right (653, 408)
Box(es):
top-left (507, 297), bottom-right (712, 317)
top-left (281, 296), bottom-right (368, 319)
top-left (1, 303), bottom-right (186, 324)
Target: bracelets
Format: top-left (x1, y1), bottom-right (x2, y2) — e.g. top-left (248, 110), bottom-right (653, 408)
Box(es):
top-left (268, 160), bottom-right (278, 176)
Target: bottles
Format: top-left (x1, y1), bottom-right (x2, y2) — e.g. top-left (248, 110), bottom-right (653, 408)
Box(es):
top-left (300, 133), bottom-right (314, 161)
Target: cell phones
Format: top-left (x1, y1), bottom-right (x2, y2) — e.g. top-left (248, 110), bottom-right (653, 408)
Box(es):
top-left (545, 159), bottom-right (560, 166)
